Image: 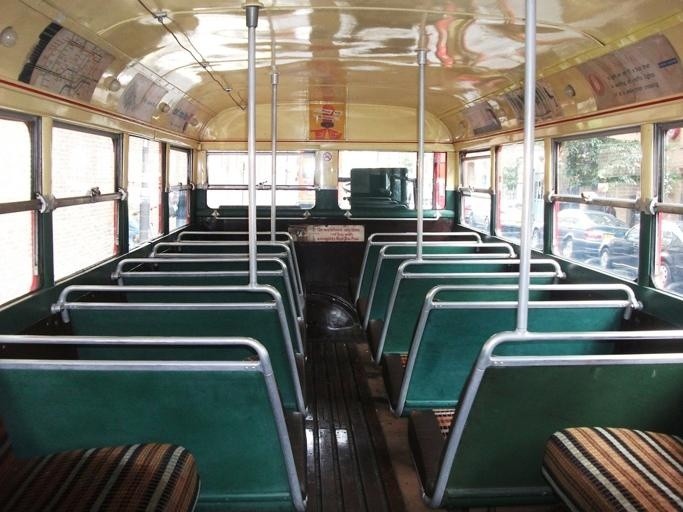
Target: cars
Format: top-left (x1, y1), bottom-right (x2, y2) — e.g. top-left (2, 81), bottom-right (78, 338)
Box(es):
top-left (598, 220), bottom-right (683, 288)
top-left (469, 204), bottom-right (524, 237)
top-left (532, 209), bottom-right (629, 262)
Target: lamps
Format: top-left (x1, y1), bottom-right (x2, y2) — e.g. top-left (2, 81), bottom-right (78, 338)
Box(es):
top-left (564, 84), bottom-right (575, 97)
top-left (0, 26), bottom-right (18, 48)
top-left (109, 79), bottom-right (170, 114)
